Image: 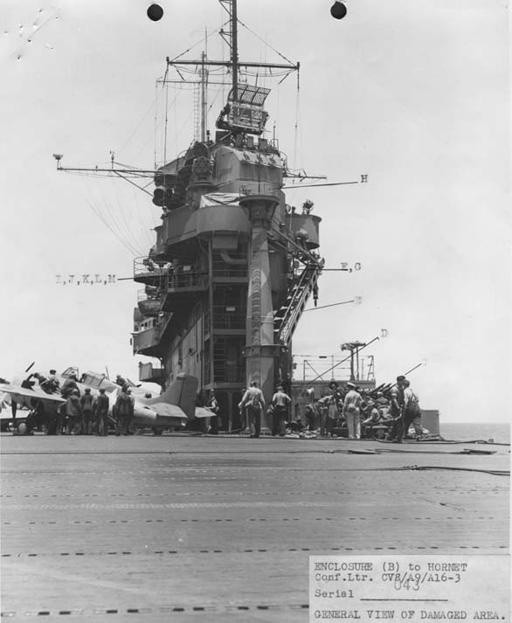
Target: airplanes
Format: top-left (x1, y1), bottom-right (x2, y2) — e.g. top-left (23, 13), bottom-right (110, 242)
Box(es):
top-left (1, 367), bottom-right (216, 435)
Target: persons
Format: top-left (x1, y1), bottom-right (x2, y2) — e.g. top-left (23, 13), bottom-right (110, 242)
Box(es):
top-left (237, 374), bottom-right (423, 444)
top-left (26, 370), bottom-right (135, 437)
top-left (194, 388), bottom-right (220, 434)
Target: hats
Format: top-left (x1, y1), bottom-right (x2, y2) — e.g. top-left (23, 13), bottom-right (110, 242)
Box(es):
top-left (71, 388), bottom-right (105, 393)
top-left (346, 382), bottom-right (355, 387)
top-left (368, 400), bottom-right (375, 406)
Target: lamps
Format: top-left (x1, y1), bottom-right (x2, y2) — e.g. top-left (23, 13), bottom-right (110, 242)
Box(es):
top-left (53, 154), bottom-right (63, 168)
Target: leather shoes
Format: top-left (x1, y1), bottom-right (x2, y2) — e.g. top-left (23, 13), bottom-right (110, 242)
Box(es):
top-left (394, 440), bottom-right (404, 443)
top-left (416, 435), bottom-right (421, 441)
